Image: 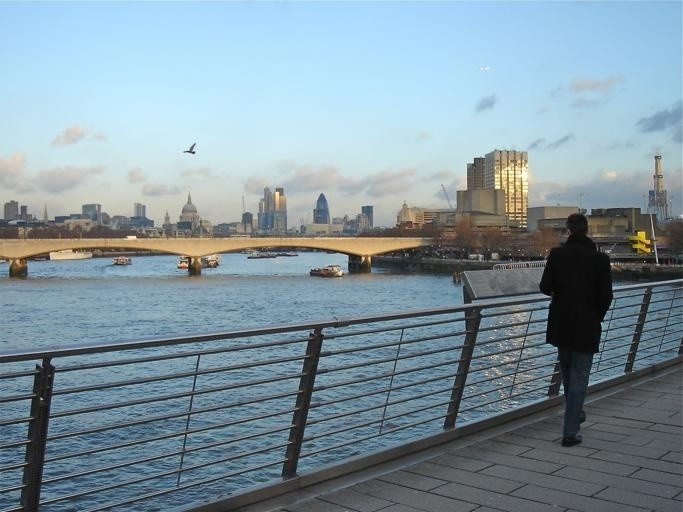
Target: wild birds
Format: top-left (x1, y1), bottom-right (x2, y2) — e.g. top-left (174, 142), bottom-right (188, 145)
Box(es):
top-left (183, 142), bottom-right (196, 154)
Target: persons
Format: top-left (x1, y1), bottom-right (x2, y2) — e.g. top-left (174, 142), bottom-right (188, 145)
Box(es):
top-left (403, 243), bottom-right (467, 260)
top-left (538, 212), bottom-right (614, 447)
top-left (453, 271), bottom-right (462, 285)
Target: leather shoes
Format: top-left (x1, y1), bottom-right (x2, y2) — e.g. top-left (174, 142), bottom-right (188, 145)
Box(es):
top-left (562, 412), bottom-right (585, 447)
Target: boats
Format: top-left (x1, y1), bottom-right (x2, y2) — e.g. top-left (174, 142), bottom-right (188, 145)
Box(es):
top-left (247, 251), bottom-right (297, 258)
top-left (178, 256), bottom-right (189, 269)
top-left (113, 256), bottom-right (128, 265)
top-left (310, 265), bottom-right (343, 277)
top-left (201, 256), bottom-right (219, 268)
